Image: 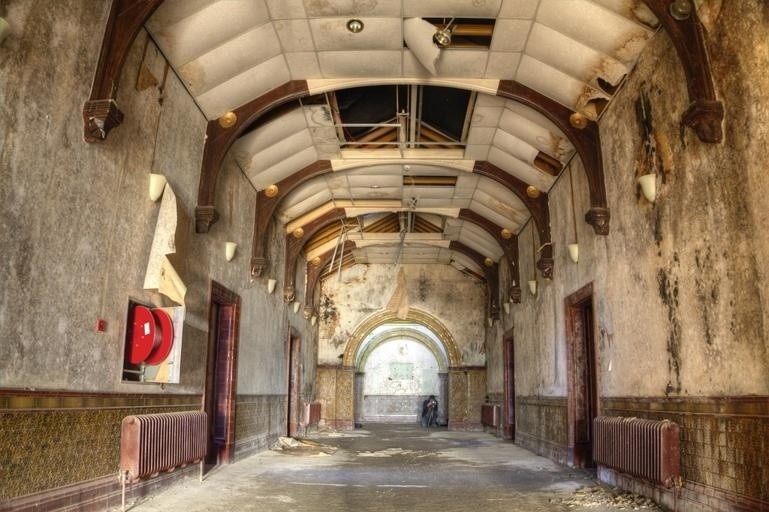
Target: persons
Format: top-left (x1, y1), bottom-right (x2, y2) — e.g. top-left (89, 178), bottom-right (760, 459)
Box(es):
top-left (422, 395), bottom-right (438, 427)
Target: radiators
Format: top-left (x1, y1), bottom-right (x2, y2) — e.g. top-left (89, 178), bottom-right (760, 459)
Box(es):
top-left (591, 414), bottom-right (684, 511)
top-left (481, 405), bottom-right (500, 438)
top-left (118, 408), bottom-right (208, 511)
